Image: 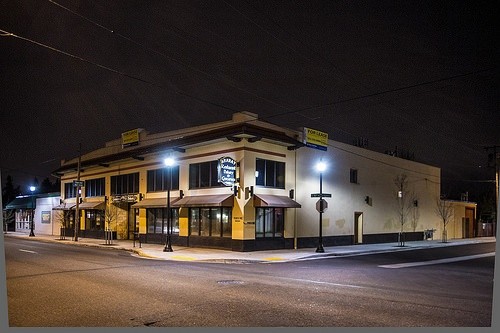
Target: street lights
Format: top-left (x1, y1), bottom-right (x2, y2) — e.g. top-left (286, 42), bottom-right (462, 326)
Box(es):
top-left (29, 185), bottom-right (36, 236)
top-left (162, 156), bottom-right (175, 252)
top-left (314, 155), bottom-right (328, 252)
